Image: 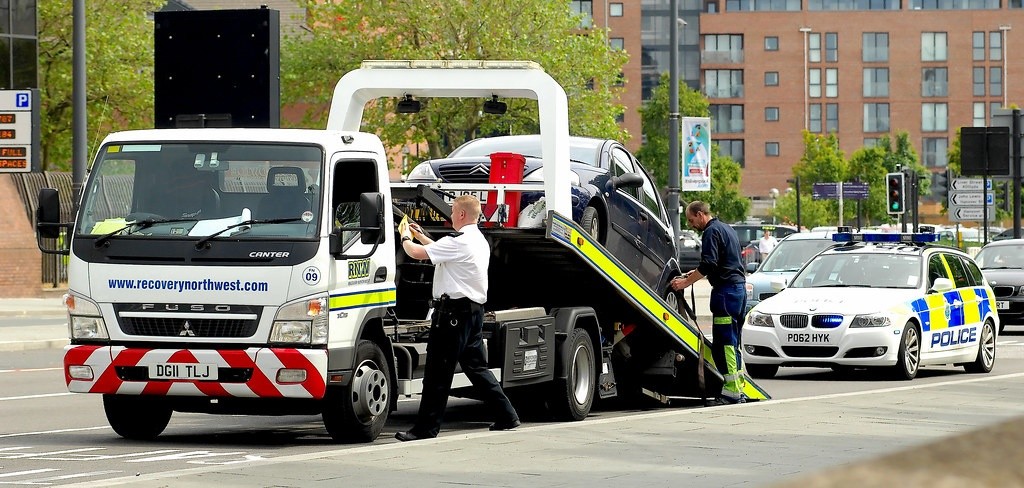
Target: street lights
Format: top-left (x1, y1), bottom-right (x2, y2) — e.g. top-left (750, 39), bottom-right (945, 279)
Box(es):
top-left (787, 176), bottom-right (801, 232)
top-left (768, 187), bottom-right (779, 223)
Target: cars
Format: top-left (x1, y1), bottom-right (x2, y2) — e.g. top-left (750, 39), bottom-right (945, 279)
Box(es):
top-left (678, 225), bottom-right (1001, 381)
top-left (405, 135), bottom-right (685, 319)
top-left (974, 228), bottom-right (1024, 333)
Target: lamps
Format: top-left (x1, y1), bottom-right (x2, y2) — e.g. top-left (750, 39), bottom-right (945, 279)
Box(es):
top-left (483, 95), bottom-right (508, 114)
top-left (396, 94), bottom-right (422, 115)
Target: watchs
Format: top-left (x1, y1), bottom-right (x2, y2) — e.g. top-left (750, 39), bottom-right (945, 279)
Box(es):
top-left (402, 236), bottom-right (411, 242)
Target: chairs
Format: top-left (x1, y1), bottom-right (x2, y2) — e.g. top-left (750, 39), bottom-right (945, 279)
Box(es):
top-left (254, 166), bottom-right (314, 223)
top-left (159, 170), bottom-right (222, 217)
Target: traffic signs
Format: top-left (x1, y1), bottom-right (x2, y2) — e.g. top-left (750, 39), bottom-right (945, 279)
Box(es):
top-left (948, 206), bottom-right (996, 222)
top-left (950, 178), bottom-right (992, 191)
top-left (948, 190), bottom-right (995, 207)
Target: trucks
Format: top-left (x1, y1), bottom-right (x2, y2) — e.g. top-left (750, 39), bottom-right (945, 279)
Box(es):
top-left (35, 60), bottom-right (771, 443)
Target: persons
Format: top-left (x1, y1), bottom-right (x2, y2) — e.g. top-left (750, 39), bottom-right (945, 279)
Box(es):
top-left (395, 196), bottom-right (522, 442)
top-left (759, 229), bottom-right (778, 263)
top-left (671, 201), bottom-right (748, 407)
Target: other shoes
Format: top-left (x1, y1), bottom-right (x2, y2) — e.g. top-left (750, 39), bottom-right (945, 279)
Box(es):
top-left (741, 395), bottom-right (747, 404)
top-left (393, 430), bottom-right (423, 441)
top-left (489, 420), bottom-right (521, 431)
top-left (704, 395), bottom-right (742, 406)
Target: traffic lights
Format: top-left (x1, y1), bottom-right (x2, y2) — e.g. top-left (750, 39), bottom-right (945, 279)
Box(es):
top-left (886, 173), bottom-right (905, 215)
